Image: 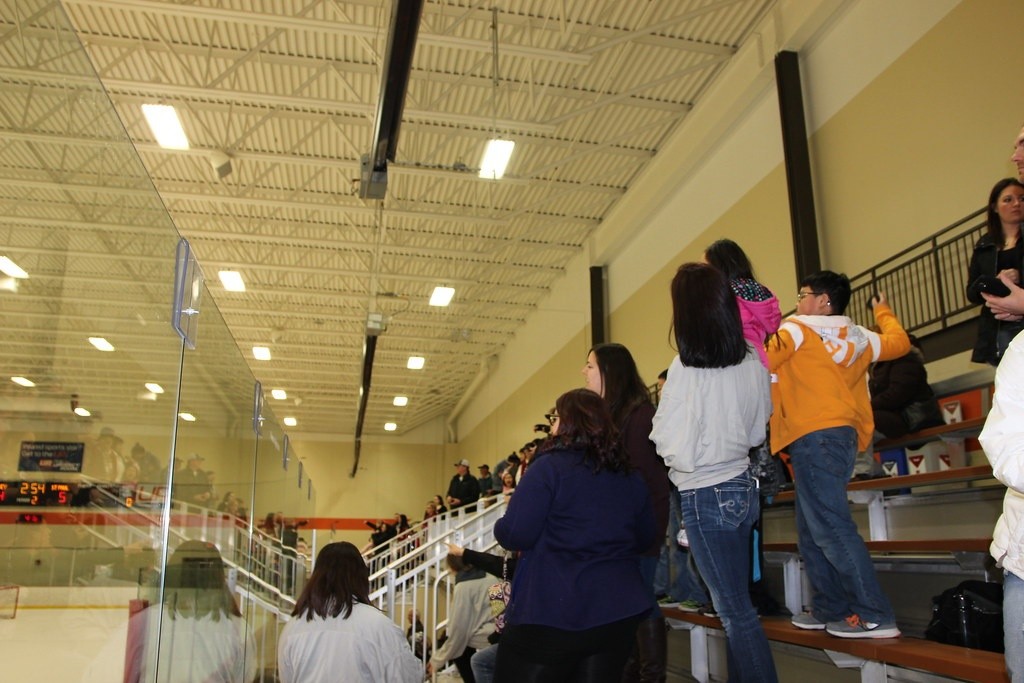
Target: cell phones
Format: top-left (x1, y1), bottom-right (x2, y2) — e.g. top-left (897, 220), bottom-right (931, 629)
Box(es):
top-left (971, 274), bottom-right (1011, 298)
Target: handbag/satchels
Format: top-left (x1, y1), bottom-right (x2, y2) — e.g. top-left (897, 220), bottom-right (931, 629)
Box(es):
top-left (748, 432), bottom-right (795, 510)
top-left (489, 581), bottom-right (512, 634)
top-left (924, 580), bottom-right (1004, 653)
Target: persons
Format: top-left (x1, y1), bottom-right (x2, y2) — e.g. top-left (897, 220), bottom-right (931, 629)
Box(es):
top-left (421, 495), bottom-right (447, 561)
top-left (765, 268), bottom-right (948, 638)
top-left (446, 423), bottom-right (551, 518)
top-left (703, 239), bottom-right (783, 501)
top-left (278, 541), bottom-right (424, 683)
top-left (653, 262), bottom-right (778, 682)
top-left (407, 609), bottom-right (431, 663)
top-left (966, 126), bottom-right (1024, 367)
top-left (1, 359), bottom-right (308, 607)
top-left (492, 388), bottom-right (652, 682)
top-left (98, 540), bottom-right (257, 682)
top-left (362, 513), bottom-right (420, 600)
top-left (426, 542), bottom-right (519, 683)
top-left (581, 342), bottom-right (668, 682)
top-left (978, 330), bottom-right (1024, 683)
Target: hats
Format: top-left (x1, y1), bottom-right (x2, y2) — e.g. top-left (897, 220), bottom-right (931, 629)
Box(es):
top-left (478, 465), bottom-right (489, 469)
top-left (455, 459), bottom-right (469, 467)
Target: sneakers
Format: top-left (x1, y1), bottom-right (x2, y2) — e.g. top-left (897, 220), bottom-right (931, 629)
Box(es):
top-left (679, 600), bottom-right (708, 612)
top-left (657, 595), bottom-right (681, 608)
top-left (791, 611), bottom-right (826, 629)
top-left (826, 614), bottom-right (901, 638)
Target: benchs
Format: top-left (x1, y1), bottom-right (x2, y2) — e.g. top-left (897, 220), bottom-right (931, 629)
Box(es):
top-left (658, 397), bottom-right (1016, 683)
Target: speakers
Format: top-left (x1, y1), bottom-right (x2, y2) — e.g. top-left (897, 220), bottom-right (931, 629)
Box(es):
top-left (212, 151), bottom-right (232, 178)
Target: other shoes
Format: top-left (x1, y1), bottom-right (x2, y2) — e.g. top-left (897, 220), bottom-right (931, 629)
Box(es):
top-left (704, 608), bottom-right (719, 617)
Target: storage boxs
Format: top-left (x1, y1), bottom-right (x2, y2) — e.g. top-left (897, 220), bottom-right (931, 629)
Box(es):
top-left (878, 384), bottom-right (1005, 497)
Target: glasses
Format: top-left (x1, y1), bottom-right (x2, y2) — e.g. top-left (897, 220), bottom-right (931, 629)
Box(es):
top-left (797, 291), bottom-right (819, 302)
top-left (545, 414), bottom-right (560, 426)
